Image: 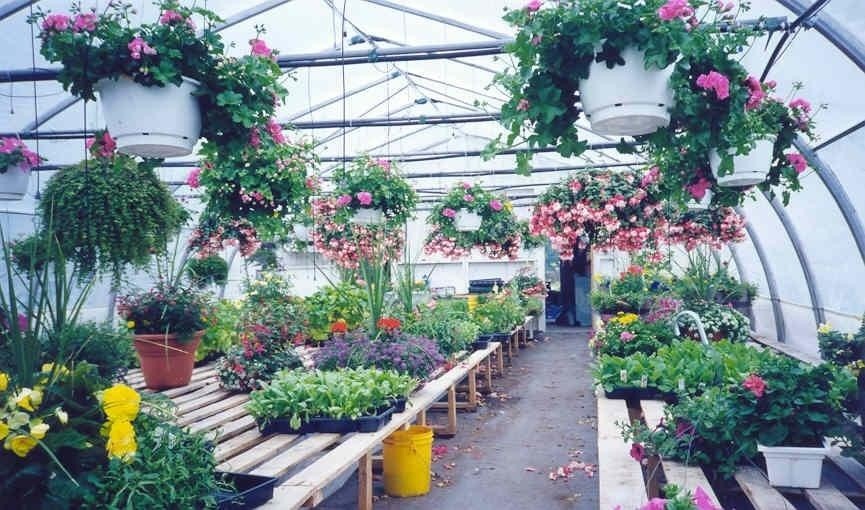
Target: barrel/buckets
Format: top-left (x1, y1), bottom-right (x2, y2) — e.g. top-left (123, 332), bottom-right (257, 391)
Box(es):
top-left (468, 279), bottom-right (502, 294)
top-left (384, 426), bottom-right (433, 498)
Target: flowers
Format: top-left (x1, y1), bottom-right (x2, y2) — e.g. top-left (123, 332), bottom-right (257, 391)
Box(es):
top-left (0, 0), bottom-right (547, 510)
top-left (418, 0), bottom-right (865, 510)
